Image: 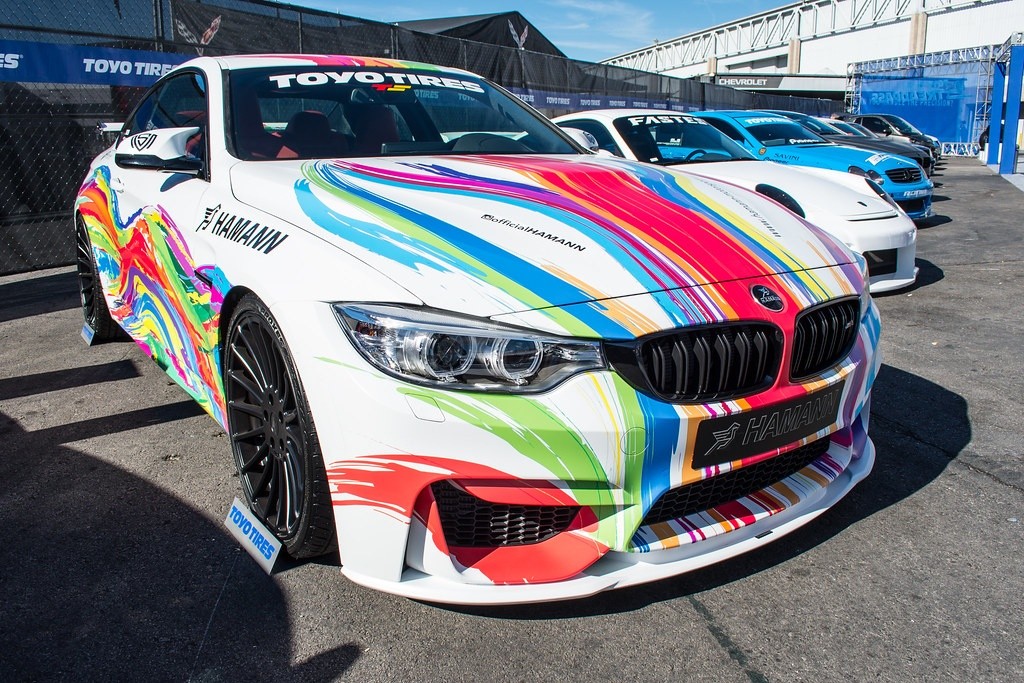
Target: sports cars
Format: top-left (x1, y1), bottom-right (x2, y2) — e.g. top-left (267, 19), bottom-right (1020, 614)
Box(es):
top-left (549, 107), bottom-right (919, 296)
top-left (607, 108), bottom-right (937, 224)
top-left (71, 52), bottom-right (885, 609)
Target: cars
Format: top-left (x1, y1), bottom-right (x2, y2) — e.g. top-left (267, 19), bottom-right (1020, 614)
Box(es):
top-left (758, 108), bottom-right (932, 181)
top-left (804, 111), bottom-right (942, 174)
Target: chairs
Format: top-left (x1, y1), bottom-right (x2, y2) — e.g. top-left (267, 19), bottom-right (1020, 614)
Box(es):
top-left (281, 103), bottom-right (400, 162)
